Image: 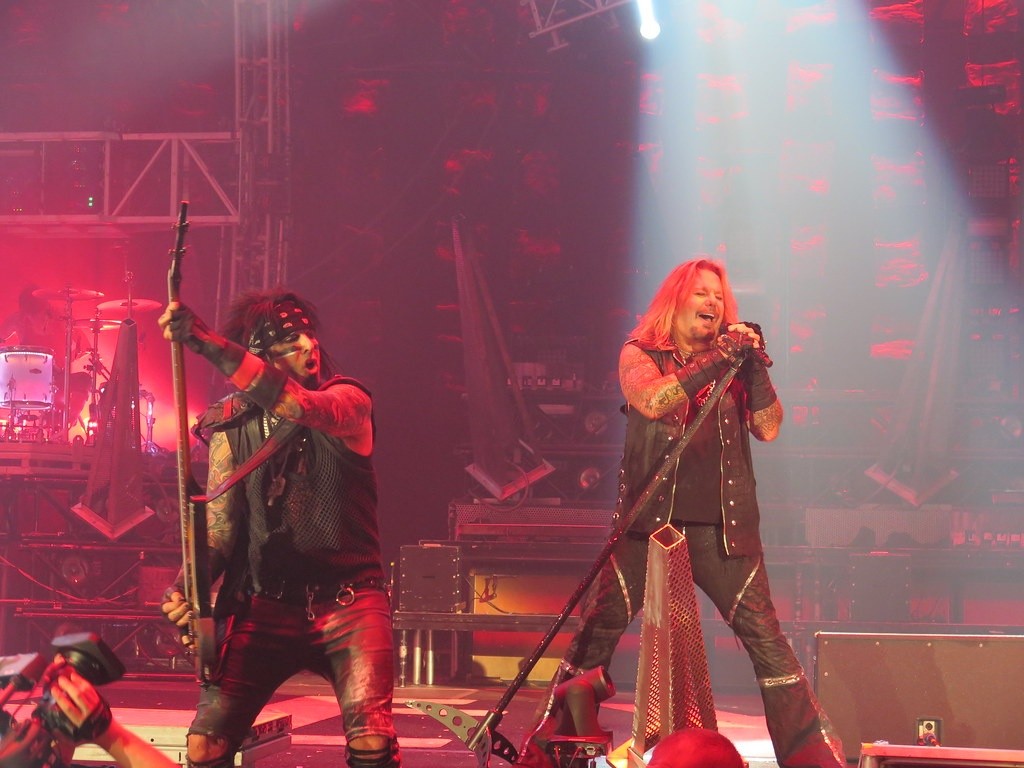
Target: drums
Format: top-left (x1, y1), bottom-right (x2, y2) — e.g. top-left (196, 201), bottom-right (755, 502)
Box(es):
top-left (0, 346), bottom-right (55, 410)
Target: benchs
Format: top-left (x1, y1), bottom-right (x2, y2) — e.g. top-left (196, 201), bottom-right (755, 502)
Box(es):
top-left (391, 390), bottom-right (1024, 704)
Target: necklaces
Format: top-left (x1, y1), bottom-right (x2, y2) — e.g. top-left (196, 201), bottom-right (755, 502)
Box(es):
top-left (678, 348), bottom-right (711, 358)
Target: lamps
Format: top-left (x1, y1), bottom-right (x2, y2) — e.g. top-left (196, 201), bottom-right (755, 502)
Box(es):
top-left (863, 453), bottom-right (960, 509)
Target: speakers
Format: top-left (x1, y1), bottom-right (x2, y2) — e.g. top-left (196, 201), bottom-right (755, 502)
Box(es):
top-left (811, 631), bottom-right (1024, 762)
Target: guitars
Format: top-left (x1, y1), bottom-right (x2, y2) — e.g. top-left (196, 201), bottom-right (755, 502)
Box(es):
top-left (168, 201), bottom-right (218, 689)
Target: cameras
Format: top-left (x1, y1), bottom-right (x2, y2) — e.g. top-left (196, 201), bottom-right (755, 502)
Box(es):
top-left (29, 633), bottom-right (125, 740)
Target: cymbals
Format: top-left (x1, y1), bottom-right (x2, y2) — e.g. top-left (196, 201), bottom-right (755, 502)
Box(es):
top-left (32, 288), bottom-right (104, 300)
top-left (96, 298), bottom-right (162, 312)
top-left (72, 319), bottom-right (122, 331)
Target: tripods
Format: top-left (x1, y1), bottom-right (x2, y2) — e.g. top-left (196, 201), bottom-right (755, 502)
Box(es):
top-left (407, 353), bottom-right (752, 768)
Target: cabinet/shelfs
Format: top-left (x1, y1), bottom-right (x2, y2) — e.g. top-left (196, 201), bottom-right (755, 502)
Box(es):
top-left (0, 462), bottom-right (209, 678)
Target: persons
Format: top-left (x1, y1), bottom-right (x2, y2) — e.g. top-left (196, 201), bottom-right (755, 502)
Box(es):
top-left (646, 727), bottom-right (744, 768)
top-left (47, 670), bottom-right (178, 768)
top-left (0, 283), bottom-right (92, 433)
top-left (517, 259), bottom-right (851, 768)
top-left (157, 290), bottom-right (403, 768)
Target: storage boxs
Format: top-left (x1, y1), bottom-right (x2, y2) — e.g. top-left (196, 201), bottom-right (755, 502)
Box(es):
top-left (399, 544), bottom-right (476, 614)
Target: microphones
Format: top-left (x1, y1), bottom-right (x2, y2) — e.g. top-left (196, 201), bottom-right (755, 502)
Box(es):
top-left (719, 322), bottom-right (773, 368)
